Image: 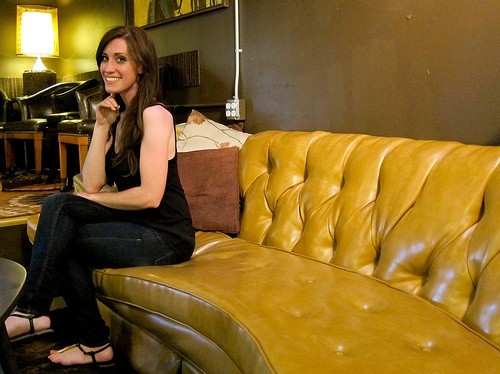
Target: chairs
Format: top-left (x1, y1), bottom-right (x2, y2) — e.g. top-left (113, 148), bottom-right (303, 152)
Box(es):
top-left (4, 77), bottom-right (97, 184)
top-left (0, 88), bottom-right (13, 179)
top-left (47, 67), bottom-right (171, 192)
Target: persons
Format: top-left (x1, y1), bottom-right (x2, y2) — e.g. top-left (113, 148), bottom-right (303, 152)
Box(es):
top-left (0, 26), bottom-right (195, 368)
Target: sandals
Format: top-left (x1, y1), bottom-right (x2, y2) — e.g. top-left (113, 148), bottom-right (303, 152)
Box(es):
top-left (0, 315), bottom-right (54, 348)
top-left (48, 343), bottom-right (115, 371)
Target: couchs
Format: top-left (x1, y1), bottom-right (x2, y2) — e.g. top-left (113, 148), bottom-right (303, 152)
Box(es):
top-left (26, 123), bottom-right (500, 374)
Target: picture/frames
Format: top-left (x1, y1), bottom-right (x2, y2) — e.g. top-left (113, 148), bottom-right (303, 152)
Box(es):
top-left (123, 0), bottom-right (229, 30)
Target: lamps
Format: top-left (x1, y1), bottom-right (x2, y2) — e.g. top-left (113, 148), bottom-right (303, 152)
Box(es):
top-left (16, 4), bottom-right (60, 72)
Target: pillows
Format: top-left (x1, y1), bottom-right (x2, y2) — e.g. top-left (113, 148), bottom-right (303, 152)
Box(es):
top-left (177, 145), bottom-right (240, 234)
top-left (176, 109), bottom-right (252, 152)
